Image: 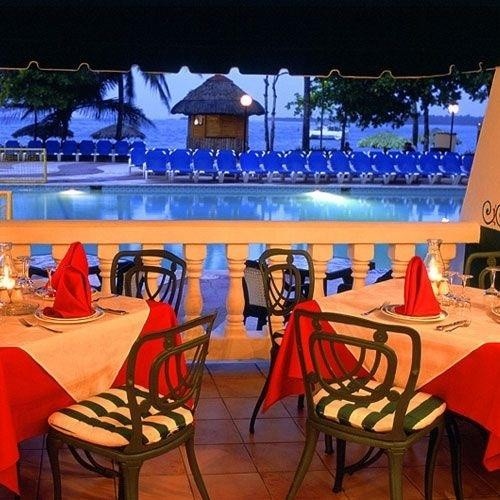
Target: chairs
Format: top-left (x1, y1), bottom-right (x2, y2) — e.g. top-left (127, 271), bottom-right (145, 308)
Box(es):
top-left (247, 249), bottom-right (316, 435)
top-left (464, 252), bottom-right (500, 290)
top-left (284, 309), bottom-right (449, 500)
top-left (109, 248), bottom-right (188, 314)
top-left (49, 313), bottom-right (217, 500)
top-left (0, 138), bottom-right (475, 185)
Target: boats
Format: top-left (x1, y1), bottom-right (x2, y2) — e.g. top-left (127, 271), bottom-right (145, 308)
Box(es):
top-left (310, 121), bottom-right (351, 140)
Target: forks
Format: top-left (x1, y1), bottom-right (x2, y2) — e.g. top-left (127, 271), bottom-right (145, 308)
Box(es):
top-left (362, 300), bottom-right (390, 316)
top-left (19, 317), bottom-right (62, 334)
top-left (92, 301), bottom-right (125, 315)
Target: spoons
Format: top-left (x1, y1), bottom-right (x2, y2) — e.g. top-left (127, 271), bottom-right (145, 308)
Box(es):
top-left (445, 321), bottom-right (472, 333)
top-left (92, 294), bottom-right (118, 303)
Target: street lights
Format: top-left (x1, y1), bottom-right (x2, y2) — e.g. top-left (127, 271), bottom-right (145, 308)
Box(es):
top-left (240, 94), bottom-right (253, 152)
top-left (447, 103), bottom-right (460, 152)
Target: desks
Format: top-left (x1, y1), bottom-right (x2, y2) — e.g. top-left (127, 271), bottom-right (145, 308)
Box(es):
top-left (0, 272), bottom-right (191, 500)
top-left (313, 275), bottom-right (500, 500)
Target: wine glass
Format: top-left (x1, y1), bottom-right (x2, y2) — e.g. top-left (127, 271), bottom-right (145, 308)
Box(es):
top-left (0, 241), bottom-right (34, 309)
top-left (481, 265), bottom-right (500, 318)
top-left (453, 273), bottom-right (475, 319)
top-left (439, 270), bottom-right (458, 308)
top-left (39, 263), bottom-right (58, 307)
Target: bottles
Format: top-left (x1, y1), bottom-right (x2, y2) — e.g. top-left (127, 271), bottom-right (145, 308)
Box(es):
top-left (423, 239), bottom-right (444, 284)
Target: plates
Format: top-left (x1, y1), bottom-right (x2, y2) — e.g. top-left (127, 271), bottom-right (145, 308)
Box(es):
top-left (381, 305), bottom-right (449, 321)
top-left (34, 305), bottom-right (106, 323)
top-left (34, 286), bottom-right (97, 300)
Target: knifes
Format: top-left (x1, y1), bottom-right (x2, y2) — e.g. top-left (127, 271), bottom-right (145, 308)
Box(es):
top-left (436, 319), bottom-right (466, 331)
